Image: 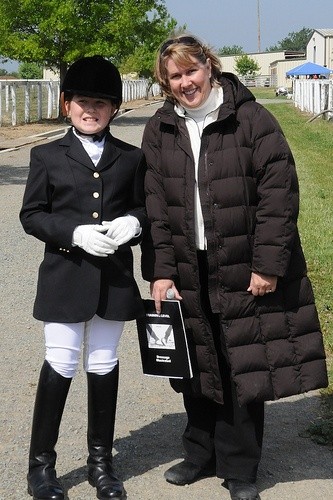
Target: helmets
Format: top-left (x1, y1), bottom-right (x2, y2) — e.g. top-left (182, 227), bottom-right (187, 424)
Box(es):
top-left (62, 55), bottom-right (123, 109)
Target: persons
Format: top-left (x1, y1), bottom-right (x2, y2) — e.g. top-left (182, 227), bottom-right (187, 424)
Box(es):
top-left (19, 56), bottom-right (150, 500)
top-left (138, 37), bottom-right (328, 500)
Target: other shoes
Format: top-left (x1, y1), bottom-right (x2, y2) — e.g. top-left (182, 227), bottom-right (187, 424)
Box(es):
top-left (164, 460), bottom-right (205, 486)
top-left (227, 478), bottom-right (258, 500)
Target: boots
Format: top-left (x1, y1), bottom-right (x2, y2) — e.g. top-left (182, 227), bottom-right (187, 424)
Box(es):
top-left (27, 360), bottom-right (73, 500)
top-left (86, 359), bottom-right (127, 500)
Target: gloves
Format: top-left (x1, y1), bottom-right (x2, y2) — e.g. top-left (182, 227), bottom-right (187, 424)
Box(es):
top-left (71, 224), bottom-right (119, 257)
top-left (101, 216), bottom-right (141, 245)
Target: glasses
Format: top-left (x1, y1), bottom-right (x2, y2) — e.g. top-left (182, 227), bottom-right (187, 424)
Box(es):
top-left (160, 36), bottom-right (201, 55)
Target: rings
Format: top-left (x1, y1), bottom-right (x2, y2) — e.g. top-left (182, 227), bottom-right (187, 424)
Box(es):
top-left (266, 289), bottom-right (272, 293)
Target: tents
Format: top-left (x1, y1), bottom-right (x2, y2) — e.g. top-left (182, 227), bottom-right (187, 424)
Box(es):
top-left (285, 61), bottom-right (333, 94)
